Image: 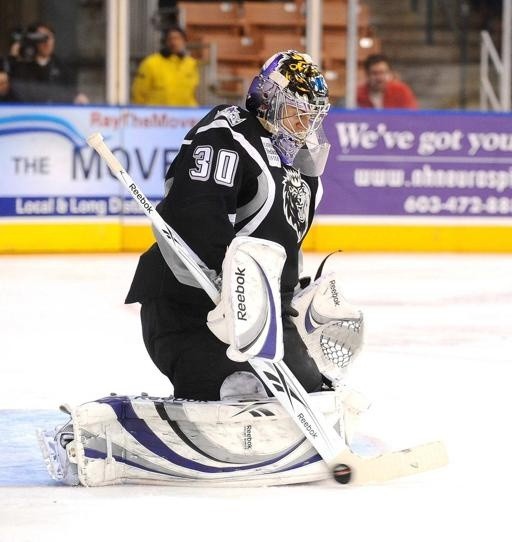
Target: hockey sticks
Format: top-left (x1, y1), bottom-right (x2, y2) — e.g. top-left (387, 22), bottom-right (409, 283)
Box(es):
top-left (84, 132), bottom-right (448, 485)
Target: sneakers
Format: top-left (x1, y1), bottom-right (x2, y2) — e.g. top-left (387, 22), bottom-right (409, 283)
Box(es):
top-left (51, 401), bottom-right (80, 487)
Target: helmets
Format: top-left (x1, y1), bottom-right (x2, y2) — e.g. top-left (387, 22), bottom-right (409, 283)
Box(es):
top-left (244, 48), bottom-right (333, 179)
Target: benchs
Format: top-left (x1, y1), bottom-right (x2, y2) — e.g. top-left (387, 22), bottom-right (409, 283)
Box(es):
top-left (177, 0), bottom-right (381, 109)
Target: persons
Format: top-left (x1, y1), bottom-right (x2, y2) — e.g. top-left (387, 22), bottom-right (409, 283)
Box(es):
top-left (130, 24), bottom-right (201, 108)
top-left (356, 51), bottom-right (420, 110)
top-left (7, 20), bottom-right (90, 106)
top-left (46, 49), bottom-right (365, 484)
